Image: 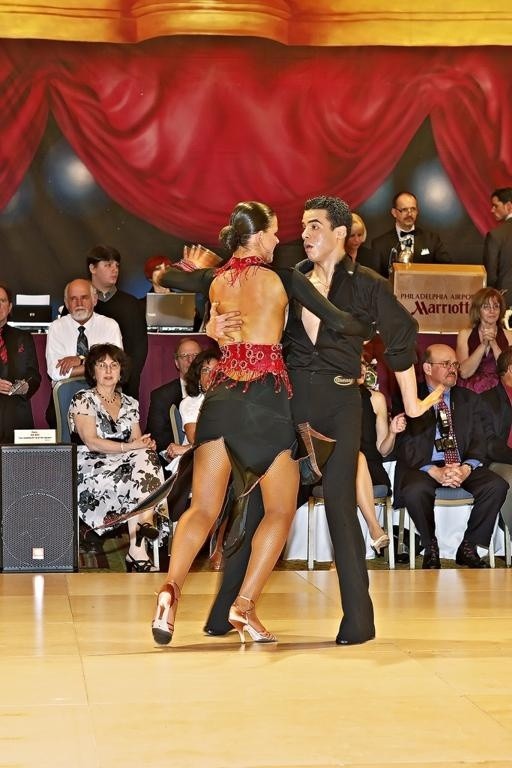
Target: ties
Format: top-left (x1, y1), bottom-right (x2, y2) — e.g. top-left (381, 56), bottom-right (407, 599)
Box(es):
top-left (76, 326), bottom-right (88, 355)
top-left (0, 328), bottom-right (9, 364)
top-left (438, 396), bottom-right (460, 462)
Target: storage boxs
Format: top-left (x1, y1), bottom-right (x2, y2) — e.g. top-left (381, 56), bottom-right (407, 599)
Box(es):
top-left (389, 260), bottom-right (489, 331)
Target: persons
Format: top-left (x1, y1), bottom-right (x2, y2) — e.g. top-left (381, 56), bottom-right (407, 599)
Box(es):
top-left (44, 275), bottom-right (127, 445)
top-left (65, 340), bottom-right (171, 574)
top-left (202, 196), bottom-right (445, 645)
top-left (132, 250), bottom-right (190, 343)
top-left (140, 333), bottom-right (228, 574)
top-left (150, 199), bottom-right (374, 645)
top-left (341, 191), bottom-right (511, 570)
top-left (72, 248), bottom-right (138, 390)
top-left (0, 286), bottom-right (40, 453)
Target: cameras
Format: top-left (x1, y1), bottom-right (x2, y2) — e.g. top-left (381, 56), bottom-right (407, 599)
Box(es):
top-left (433, 409), bottom-right (456, 452)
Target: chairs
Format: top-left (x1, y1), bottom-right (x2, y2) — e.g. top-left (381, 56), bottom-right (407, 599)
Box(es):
top-left (503, 518), bottom-right (512, 566)
top-left (51, 377), bottom-right (162, 571)
top-left (168, 403), bottom-right (185, 446)
top-left (283, 484), bottom-right (399, 571)
top-left (398, 480), bottom-right (503, 569)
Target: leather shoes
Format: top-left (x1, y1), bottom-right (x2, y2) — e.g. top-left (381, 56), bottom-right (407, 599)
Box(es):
top-left (456, 543), bottom-right (490, 568)
top-left (422, 536), bottom-right (441, 569)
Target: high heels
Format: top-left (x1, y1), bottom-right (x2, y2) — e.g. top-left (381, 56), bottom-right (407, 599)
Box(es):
top-left (135, 522), bottom-right (159, 547)
top-left (152, 582), bottom-right (180, 644)
top-left (370, 535), bottom-right (389, 554)
top-left (226, 596), bottom-right (277, 644)
top-left (125, 553), bottom-right (159, 573)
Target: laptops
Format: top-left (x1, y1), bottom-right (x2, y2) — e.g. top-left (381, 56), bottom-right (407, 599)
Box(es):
top-left (145, 292), bottom-right (196, 332)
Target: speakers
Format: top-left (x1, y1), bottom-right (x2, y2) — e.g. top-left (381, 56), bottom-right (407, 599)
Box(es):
top-left (1, 443), bottom-right (73, 571)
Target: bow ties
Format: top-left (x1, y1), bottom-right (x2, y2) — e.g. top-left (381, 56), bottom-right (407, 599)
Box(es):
top-left (400, 230), bottom-right (417, 236)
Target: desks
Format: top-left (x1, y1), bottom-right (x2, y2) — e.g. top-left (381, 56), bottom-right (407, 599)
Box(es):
top-left (28, 331), bottom-right (511, 436)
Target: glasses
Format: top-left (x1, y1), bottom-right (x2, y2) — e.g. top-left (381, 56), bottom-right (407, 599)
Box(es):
top-left (97, 363), bottom-right (119, 370)
top-left (430, 362), bottom-right (460, 369)
top-left (483, 304), bottom-right (500, 310)
top-left (395, 207), bottom-right (419, 213)
top-left (178, 353), bottom-right (198, 360)
